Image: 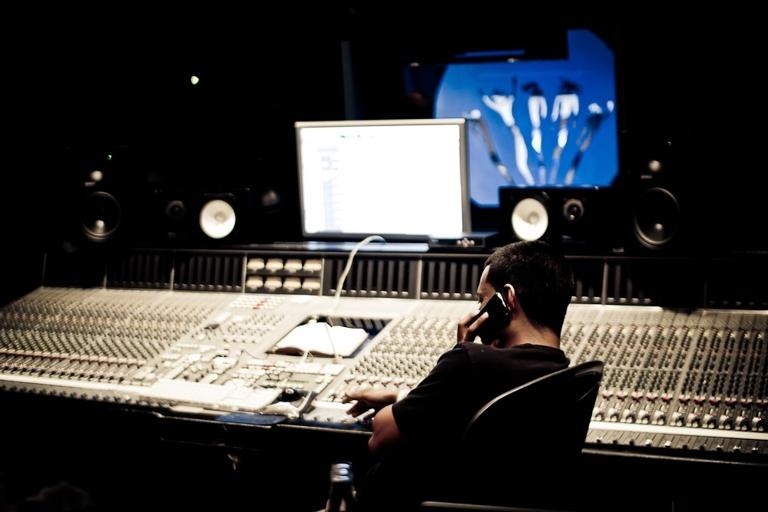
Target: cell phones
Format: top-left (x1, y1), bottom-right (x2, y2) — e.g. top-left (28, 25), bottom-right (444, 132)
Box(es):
top-left (473, 292), bottom-right (513, 344)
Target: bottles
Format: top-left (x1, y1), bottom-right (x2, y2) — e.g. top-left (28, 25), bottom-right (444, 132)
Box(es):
top-left (319, 462), bottom-right (366, 511)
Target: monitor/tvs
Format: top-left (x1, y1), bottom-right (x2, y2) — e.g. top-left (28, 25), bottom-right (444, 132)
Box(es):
top-left (292, 118), bottom-right (469, 252)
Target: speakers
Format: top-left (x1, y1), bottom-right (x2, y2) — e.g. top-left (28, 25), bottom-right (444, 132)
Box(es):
top-left (76, 146), bottom-right (250, 245)
top-left (500, 156), bottom-right (682, 256)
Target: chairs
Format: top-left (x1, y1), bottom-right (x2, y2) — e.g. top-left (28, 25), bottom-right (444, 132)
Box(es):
top-left (421, 361), bottom-right (603, 512)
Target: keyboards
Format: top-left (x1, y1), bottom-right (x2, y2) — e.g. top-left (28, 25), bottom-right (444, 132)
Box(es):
top-left (142, 379), bottom-right (282, 411)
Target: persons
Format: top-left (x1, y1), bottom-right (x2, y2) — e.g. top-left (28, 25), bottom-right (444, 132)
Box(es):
top-left (339, 240), bottom-right (572, 511)
